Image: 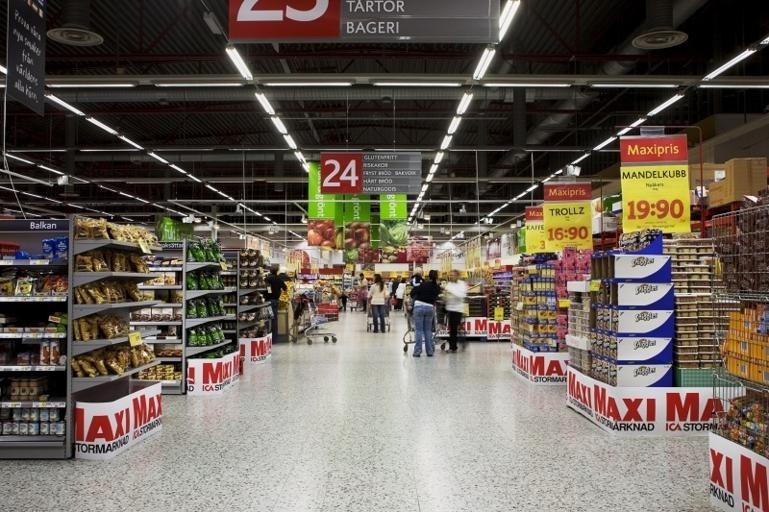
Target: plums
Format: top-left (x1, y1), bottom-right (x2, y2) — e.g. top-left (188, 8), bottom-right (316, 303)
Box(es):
top-left (345, 222), bottom-right (369, 249)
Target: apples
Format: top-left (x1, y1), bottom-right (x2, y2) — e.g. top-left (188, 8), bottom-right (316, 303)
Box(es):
top-left (308, 220), bottom-right (335, 247)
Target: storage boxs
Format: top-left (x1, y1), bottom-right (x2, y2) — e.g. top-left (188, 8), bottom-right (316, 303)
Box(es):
top-left (591, 216), bottom-right (617, 235)
top-left (707, 156), bottom-right (766, 208)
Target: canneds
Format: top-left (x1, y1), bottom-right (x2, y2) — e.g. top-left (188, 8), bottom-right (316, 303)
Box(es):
top-left (11, 379), bottom-right (50, 401)
top-left (0, 409), bottom-right (66, 436)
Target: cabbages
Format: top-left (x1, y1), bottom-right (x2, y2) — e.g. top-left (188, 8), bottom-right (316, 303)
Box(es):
top-left (379, 223), bottom-right (408, 245)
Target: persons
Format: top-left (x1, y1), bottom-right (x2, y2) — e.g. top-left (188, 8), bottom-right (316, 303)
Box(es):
top-left (438, 269), bottom-right (469, 354)
top-left (410, 269), bottom-right (442, 357)
top-left (357, 266), bottom-right (425, 334)
top-left (263, 263), bottom-right (288, 345)
top-left (338, 291), bottom-right (350, 312)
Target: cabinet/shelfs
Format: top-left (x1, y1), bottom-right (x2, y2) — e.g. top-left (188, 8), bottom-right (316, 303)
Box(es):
top-left (130, 239), bottom-right (232, 395)
top-left (220, 248), bottom-right (274, 354)
top-left (0, 218), bottom-right (164, 459)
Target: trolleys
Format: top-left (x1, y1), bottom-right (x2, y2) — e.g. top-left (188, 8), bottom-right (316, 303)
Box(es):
top-left (403, 305), bottom-right (454, 353)
top-left (288, 291), bottom-right (342, 344)
top-left (365, 299), bottom-right (392, 333)
top-left (345, 290), bottom-right (368, 313)
